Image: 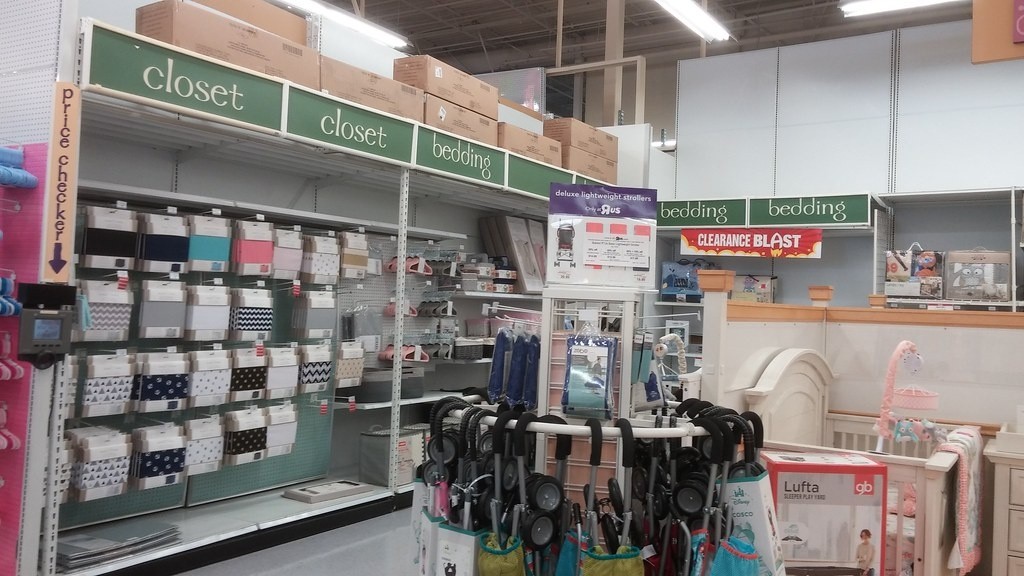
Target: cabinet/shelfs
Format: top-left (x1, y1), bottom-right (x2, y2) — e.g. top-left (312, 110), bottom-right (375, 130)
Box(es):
top-left (983, 439), bottom-right (1024, 576)
top-left (641, 193), bottom-right (893, 359)
top-left (334, 120), bottom-right (620, 514)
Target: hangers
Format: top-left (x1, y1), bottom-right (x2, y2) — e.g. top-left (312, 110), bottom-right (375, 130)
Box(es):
top-left (0, 135), bottom-right (33, 487)
top-left (377, 244), bottom-right (463, 364)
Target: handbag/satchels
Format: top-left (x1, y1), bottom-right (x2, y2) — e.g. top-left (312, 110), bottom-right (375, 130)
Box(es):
top-left (884, 241), bottom-right (945, 299)
top-left (661, 258), bottom-right (711, 298)
top-left (730, 273), bottom-right (778, 303)
top-left (945, 246), bottom-right (1011, 302)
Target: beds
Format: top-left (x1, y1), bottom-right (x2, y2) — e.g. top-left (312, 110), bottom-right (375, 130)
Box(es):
top-left (744, 346), bottom-right (982, 576)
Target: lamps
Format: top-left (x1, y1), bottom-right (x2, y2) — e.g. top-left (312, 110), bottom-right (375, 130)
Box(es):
top-left (837, 0), bottom-right (963, 19)
top-left (653, 0), bottom-right (739, 45)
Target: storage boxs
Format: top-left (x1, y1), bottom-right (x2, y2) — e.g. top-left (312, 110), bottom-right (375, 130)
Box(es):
top-left (455, 213), bottom-right (548, 362)
top-left (760, 452), bottom-right (888, 576)
top-left (135, 1), bottom-right (619, 187)
